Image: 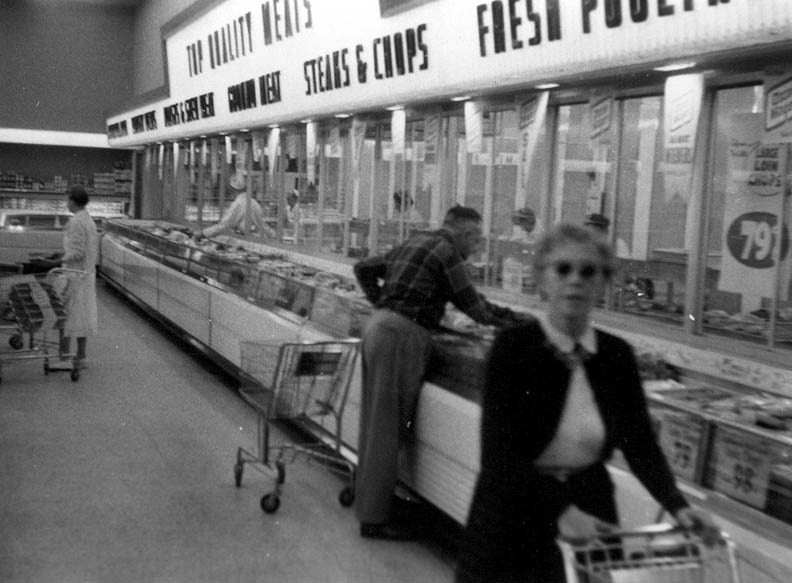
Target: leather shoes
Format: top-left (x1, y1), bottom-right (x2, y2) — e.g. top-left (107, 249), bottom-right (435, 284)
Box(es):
top-left (360, 522), bottom-right (417, 539)
top-left (388, 493), bottom-right (420, 513)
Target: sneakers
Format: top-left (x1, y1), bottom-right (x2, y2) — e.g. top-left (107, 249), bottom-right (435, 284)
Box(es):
top-left (46, 359), bottom-right (74, 370)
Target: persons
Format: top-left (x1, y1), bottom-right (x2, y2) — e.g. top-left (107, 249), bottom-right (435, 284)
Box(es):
top-left (454, 221), bottom-right (729, 583)
top-left (193, 174), bottom-right (306, 246)
top-left (354, 204), bottom-right (539, 539)
top-left (42, 187), bottom-right (99, 369)
top-left (393, 192), bottom-right (632, 271)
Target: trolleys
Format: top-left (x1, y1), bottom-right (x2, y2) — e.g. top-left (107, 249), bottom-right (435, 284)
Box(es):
top-left (234, 335), bottom-right (363, 514)
top-left (553, 524), bottom-right (744, 583)
top-left (0, 266), bottom-right (91, 383)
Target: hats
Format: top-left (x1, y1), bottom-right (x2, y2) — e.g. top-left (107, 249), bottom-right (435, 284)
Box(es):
top-left (586, 213), bottom-right (610, 226)
top-left (228, 173), bottom-right (246, 190)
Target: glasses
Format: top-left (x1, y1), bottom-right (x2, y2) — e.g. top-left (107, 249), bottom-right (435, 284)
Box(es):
top-left (541, 261), bottom-right (609, 280)
